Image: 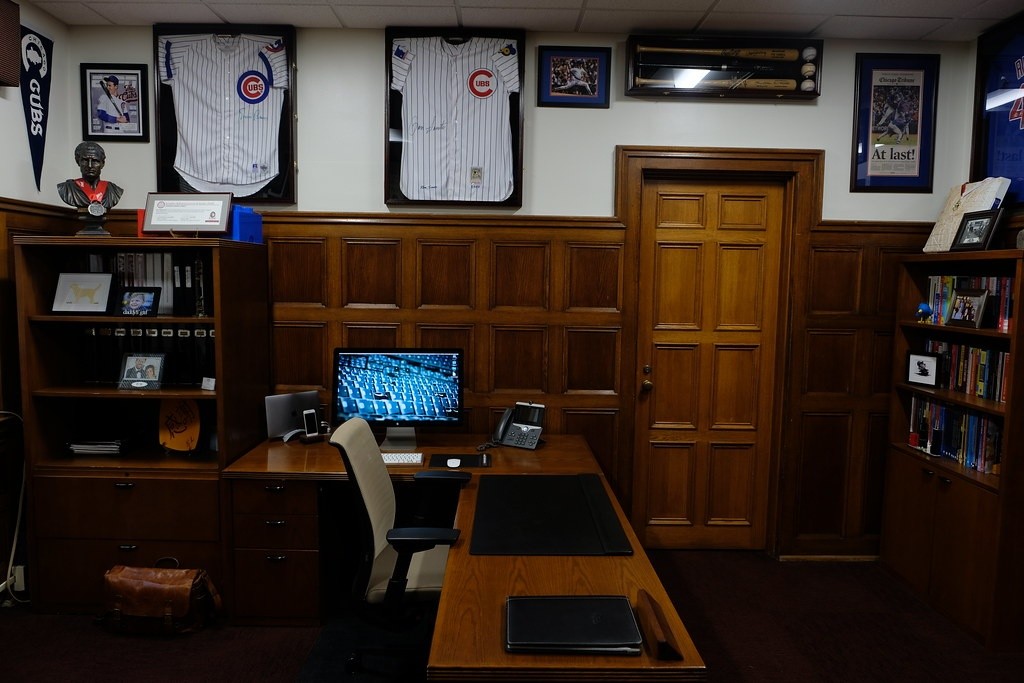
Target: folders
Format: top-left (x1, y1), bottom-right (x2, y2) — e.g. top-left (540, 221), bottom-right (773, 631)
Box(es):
top-left (65, 438), bottom-right (126, 456)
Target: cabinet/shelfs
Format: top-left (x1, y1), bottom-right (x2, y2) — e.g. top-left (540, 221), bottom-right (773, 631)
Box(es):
top-left (13, 233), bottom-right (275, 615)
top-left (878, 249), bottom-right (1024, 654)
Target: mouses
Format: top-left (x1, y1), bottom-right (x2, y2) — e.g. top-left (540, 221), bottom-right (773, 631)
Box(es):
top-left (447, 459), bottom-right (460, 467)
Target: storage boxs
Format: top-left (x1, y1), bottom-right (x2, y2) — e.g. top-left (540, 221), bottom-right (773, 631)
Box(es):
top-left (226, 206), bottom-right (262, 244)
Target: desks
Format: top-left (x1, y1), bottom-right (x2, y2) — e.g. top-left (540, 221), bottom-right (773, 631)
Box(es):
top-left (221, 434), bottom-right (709, 683)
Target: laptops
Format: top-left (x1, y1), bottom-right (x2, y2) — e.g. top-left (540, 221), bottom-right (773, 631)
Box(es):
top-left (507, 592), bottom-right (642, 656)
top-left (265, 391), bottom-right (319, 439)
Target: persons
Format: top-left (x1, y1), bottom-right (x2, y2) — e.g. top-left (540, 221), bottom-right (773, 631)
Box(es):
top-left (96, 75), bottom-right (131, 131)
top-left (873, 85), bottom-right (919, 144)
top-left (123, 293), bottom-right (150, 312)
top-left (125, 358), bottom-right (157, 380)
top-left (57, 142), bottom-right (124, 214)
top-left (552, 57), bottom-right (597, 97)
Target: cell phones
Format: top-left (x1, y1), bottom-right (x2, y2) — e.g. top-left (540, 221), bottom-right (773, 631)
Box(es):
top-left (303, 409), bottom-right (318, 437)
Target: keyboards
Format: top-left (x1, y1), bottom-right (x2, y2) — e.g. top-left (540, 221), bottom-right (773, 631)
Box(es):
top-left (381, 453), bottom-right (424, 466)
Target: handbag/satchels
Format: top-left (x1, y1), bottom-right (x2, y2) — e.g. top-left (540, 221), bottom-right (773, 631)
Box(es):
top-left (104, 556), bottom-right (223, 637)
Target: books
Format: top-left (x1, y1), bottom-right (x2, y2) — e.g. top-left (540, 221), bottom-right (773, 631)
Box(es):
top-left (67, 422), bottom-right (126, 456)
top-left (87, 250), bottom-right (206, 317)
top-left (907, 274), bottom-right (1011, 475)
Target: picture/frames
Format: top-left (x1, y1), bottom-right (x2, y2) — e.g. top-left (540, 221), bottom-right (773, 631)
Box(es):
top-left (945, 288), bottom-right (990, 329)
top-left (949, 208), bottom-right (1003, 251)
top-left (153, 22), bottom-right (298, 205)
top-left (905, 348), bottom-right (942, 388)
top-left (537, 45), bottom-right (612, 108)
top-left (850, 52), bottom-right (941, 194)
top-left (625, 26), bottom-right (824, 100)
top-left (46, 271), bottom-right (162, 319)
top-left (383, 25), bottom-right (526, 208)
top-left (118, 352), bottom-right (167, 390)
top-left (80, 63), bottom-right (150, 142)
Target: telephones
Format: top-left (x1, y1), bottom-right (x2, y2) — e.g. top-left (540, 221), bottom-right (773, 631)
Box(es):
top-left (491, 401), bottom-right (546, 450)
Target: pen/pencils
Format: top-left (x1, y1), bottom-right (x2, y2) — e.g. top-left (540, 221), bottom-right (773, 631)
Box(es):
top-left (483, 454), bottom-right (486, 464)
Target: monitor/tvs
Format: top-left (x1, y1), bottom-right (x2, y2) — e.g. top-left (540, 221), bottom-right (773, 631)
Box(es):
top-left (331, 346), bottom-right (464, 450)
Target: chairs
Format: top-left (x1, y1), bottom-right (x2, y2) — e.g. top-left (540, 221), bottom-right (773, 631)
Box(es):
top-left (329, 416), bottom-right (471, 683)
